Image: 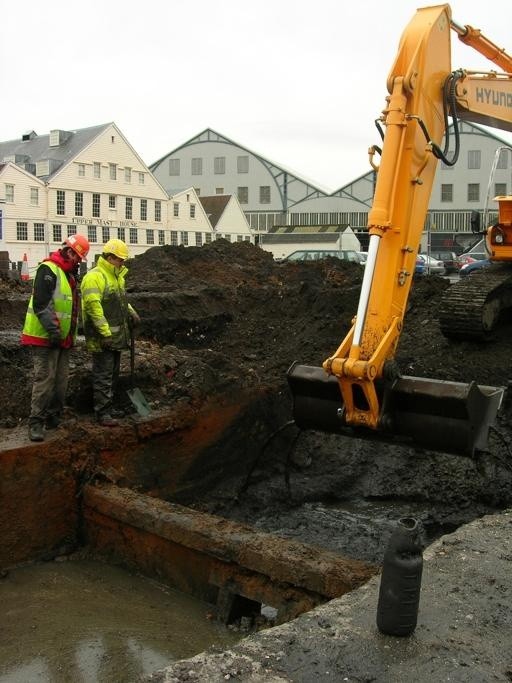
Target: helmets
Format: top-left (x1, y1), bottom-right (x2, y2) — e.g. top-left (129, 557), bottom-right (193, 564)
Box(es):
top-left (103, 239), bottom-right (129, 260)
top-left (65, 234), bottom-right (91, 261)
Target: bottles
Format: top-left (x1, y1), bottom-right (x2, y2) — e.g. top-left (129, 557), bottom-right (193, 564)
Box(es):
top-left (374, 519), bottom-right (424, 636)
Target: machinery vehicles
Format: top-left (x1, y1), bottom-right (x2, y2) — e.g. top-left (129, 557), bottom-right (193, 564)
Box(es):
top-left (287, 4), bottom-right (511, 460)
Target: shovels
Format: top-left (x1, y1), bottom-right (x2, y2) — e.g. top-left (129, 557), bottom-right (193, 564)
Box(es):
top-left (127, 316), bottom-right (150, 415)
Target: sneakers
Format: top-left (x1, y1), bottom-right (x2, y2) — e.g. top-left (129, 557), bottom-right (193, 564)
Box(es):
top-left (28, 423), bottom-right (59, 441)
top-left (98, 409), bottom-right (125, 426)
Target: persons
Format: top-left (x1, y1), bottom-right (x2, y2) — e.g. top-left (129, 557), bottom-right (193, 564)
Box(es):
top-left (81, 239), bottom-right (141, 427)
top-left (19, 235), bottom-right (91, 442)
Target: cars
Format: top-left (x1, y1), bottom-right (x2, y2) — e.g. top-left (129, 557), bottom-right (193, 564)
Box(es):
top-left (274, 250), bottom-right (491, 278)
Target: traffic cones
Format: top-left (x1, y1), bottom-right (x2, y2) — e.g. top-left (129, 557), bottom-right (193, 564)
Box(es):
top-left (21, 253), bottom-right (29, 281)
